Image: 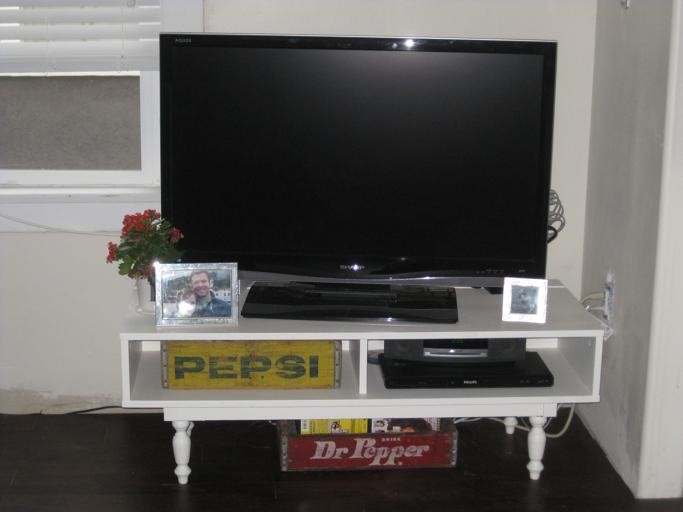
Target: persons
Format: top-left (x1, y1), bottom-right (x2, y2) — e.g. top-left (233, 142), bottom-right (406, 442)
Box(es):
top-left (189, 270), bottom-right (231, 317)
top-left (162, 288), bottom-right (197, 318)
top-left (386, 418), bottom-right (435, 433)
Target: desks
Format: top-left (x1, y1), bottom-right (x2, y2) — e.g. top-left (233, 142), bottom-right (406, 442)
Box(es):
top-left (120, 281), bottom-right (604, 485)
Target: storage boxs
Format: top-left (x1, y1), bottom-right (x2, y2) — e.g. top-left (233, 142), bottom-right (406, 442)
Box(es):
top-left (160, 341), bottom-right (342, 390)
top-left (276, 421), bottom-right (460, 473)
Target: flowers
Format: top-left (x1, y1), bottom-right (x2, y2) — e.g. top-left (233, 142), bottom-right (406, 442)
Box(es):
top-left (106, 210), bottom-right (187, 281)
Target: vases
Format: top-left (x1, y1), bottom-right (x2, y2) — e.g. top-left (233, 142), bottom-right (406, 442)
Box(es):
top-left (135, 274), bottom-right (156, 316)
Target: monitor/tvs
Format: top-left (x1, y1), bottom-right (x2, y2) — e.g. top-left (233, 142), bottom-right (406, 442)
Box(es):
top-left (159, 31), bottom-right (559, 323)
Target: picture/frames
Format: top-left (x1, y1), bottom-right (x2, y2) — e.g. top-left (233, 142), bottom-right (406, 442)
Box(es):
top-left (154, 263), bottom-right (239, 326)
top-left (502, 277), bottom-right (547, 324)
